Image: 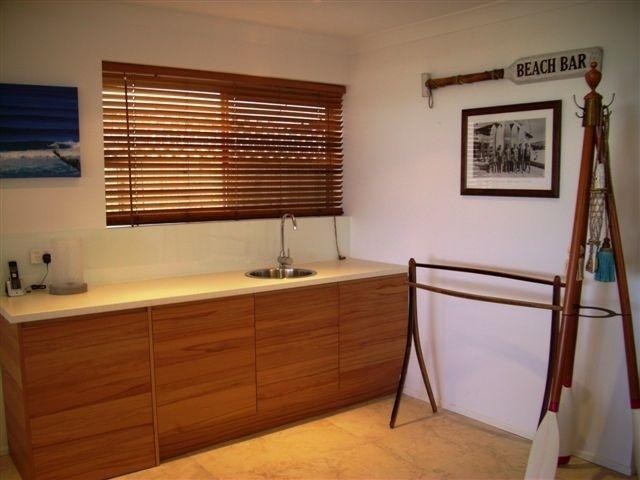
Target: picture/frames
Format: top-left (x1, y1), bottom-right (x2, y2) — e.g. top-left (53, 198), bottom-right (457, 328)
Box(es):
top-left (459, 99), bottom-right (561, 199)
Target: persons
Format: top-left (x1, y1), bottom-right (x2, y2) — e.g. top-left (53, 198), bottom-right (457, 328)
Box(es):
top-left (487, 142), bottom-right (531, 174)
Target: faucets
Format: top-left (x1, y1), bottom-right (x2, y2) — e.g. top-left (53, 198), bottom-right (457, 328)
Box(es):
top-left (281, 214), bottom-right (297, 257)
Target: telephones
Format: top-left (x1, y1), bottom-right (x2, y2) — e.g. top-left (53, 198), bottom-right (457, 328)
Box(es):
top-left (5, 261), bottom-right (23, 296)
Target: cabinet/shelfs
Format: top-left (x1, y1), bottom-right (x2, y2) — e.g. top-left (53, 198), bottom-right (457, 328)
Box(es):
top-left (256, 281), bottom-right (341, 430)
top-left (338, 274), bottom-right (410, 406)
top-left (0, 306), bottom-right (157, 478)
top-left (155, 297), bottom-right (256, 460)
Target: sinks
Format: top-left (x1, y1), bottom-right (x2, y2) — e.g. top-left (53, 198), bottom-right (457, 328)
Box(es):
top-left (245, 267), bottom-right (316, 280)
